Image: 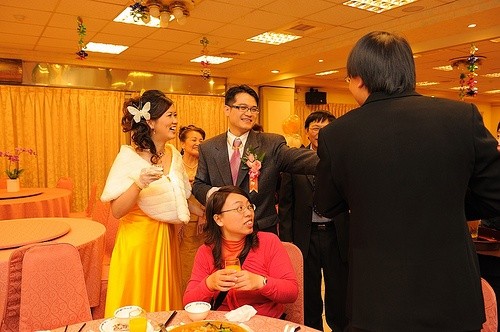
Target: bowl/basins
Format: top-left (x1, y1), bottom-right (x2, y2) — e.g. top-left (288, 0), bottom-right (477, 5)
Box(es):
top-left (184, 301), bottom-right (211, 321)
top-left (114, 306), bottom-right (142, 326)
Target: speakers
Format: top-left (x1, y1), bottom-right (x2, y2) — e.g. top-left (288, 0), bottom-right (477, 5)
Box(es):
top-left (305, 92), bottom-right (327, 105)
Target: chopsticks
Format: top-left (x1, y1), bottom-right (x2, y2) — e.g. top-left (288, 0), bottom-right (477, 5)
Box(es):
top-left (64, 323), bottom-right (86, 332)
top-left (159, 311), bottom-right (177, 332)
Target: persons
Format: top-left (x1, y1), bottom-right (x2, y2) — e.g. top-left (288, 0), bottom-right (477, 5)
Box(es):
top-left (478, 122), bottom-right (500, 332)
top-left (99, 90), bottom-right (208, 319)
top-left (177, 125), bottom-right (206, 291)
top-left (278, 110), bottom-right (346, 332)
top-left (315, 31), bottom-right (494, 332)
top-left (182, 186), bottom-right (298, 319)
top-left (192, 84), bottom-right (323, 239)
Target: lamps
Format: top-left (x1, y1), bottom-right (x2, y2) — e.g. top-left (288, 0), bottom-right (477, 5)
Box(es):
top-left (145, 0), bottom-right (195, 27)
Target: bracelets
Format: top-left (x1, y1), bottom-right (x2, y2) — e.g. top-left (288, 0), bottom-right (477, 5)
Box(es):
top-left (133, 181), bottom-right (142, 191)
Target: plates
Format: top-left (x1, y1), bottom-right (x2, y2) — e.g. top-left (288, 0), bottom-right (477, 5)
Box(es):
top-left (99, 317), bottom-right (154, 332)
top-left (159, 320), bottom-right (256, 332)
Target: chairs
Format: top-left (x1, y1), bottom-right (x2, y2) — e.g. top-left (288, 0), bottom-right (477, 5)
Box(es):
top-left (1, 178), bottom-right (121, 332)
top-left (282, 242), bottom-right (304, 326)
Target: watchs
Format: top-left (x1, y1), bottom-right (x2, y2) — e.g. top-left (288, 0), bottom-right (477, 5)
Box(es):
top-left (263, 277), bottom-right (268, 286)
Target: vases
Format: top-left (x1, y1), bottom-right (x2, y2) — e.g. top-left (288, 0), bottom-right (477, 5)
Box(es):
top-left (6, 180), bottom-right (20, 192)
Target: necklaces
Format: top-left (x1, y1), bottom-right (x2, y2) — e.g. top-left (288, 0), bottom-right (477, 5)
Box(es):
top-left (182, 160), bottom-right (200, 170)
top-left (137, 146), bottom-right (167, 170)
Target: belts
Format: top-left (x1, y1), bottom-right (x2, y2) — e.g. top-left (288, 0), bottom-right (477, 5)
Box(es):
top-left (314, 221), bottom-right (334, 232)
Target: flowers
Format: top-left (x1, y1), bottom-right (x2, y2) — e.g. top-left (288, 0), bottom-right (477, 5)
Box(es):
top-left (1, 147), bottom-right (39, 179)
top-left (242, 147), bottom-right (266, 180)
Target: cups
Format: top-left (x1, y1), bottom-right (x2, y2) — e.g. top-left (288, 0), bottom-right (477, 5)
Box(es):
top-left (467, 220), bottom-right (478, 239)
top-left (225, 258), bottom-right (241, 284)
top-left (128, 309), bottom-right (147, 332)
top-left (153, 164), bottom-right (163, 178)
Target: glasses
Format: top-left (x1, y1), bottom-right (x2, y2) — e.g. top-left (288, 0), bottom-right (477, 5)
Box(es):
top-left (216, 203), bottom-right (256, 215)
top-left (309, 127), bottom-right (324, 133)
top-left (344, 75), bottom-right (351, 84)
top-left (229, 104), bottom-right (260, 115)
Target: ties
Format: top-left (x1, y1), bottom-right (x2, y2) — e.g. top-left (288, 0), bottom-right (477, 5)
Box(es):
top-left (229, 138), bottom-right (242, 186)
top-left (312, 174), bottom-right (323, 218)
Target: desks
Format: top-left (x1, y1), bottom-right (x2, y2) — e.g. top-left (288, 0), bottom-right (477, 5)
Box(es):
top-left (0, 217), bottom-right (107, 332)
top-left (0, 188), bottom-right (72, 220)
top-left (53, 311), bottom-right (323, 332)
top-left (472, 234), bottom-right (499, 260)
